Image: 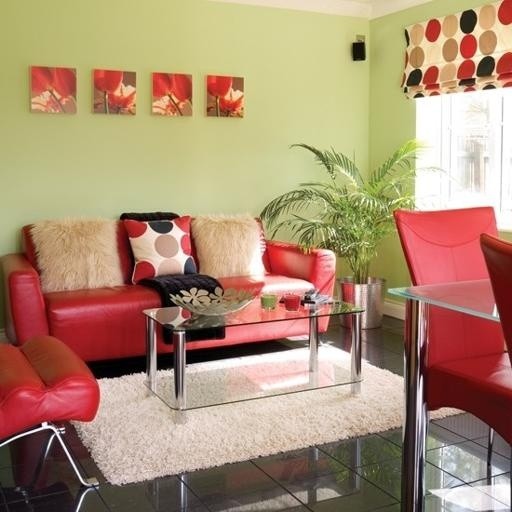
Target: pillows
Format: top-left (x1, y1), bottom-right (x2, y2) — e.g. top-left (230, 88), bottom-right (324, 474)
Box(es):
top-left (30, 218), bottom-right (123, 295)
top-left (125, 218), bottom-right (196, 280)
top-left (194, 212), bottom-right (267, 278)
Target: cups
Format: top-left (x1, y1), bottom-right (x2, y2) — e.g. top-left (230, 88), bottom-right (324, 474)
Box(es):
top-left (284, 295), bottom-right (301, 311)
top-left (260, 294), bottom-right (275, 311)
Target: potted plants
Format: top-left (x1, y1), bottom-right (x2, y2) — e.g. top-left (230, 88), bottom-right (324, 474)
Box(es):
top-left (262, 138), bottom-right (442, 330)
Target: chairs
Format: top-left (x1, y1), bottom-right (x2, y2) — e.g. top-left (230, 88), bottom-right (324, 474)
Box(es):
top-left (479, 233), bottom-right (511, 364)
top-left (394, 206), bottom-right (512, 487)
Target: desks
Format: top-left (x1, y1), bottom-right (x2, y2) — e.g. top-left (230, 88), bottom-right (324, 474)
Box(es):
top-left (388, 279), bottom-right (511, 512)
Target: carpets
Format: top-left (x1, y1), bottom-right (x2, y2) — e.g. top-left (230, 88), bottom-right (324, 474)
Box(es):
top-left (67, 342), bottom-right (471, 485)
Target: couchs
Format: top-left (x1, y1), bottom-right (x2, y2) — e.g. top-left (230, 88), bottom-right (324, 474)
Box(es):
top-left (0, 218), bottom-right (338, 364)
top-left (2, 336), bottom-right (101, 500)
